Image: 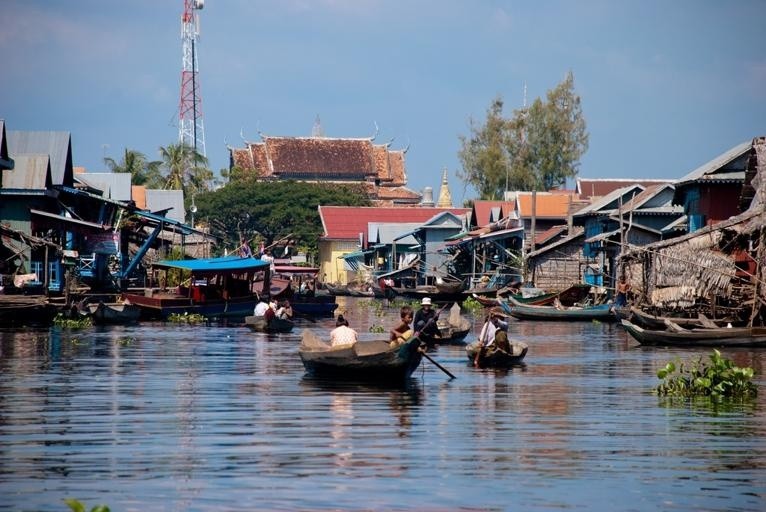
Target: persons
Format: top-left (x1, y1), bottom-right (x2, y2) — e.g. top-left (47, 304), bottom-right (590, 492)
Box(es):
top-left (412, 297), bottom-right (443, 339)
top-left (613, 275), bottom-right (633, 309)
top-left (329, 314), bottom-right (358, 347)
top-left (389, 305), bottom-right (421, 350)
top-left (260, 247), bottom-right (275, 285)
top-left (253, 278), bottom-right (319, 322)
top-left (475, 305), bottom-right (512, 355)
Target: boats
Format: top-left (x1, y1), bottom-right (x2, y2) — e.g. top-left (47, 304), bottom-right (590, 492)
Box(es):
top-left (85, 298), bottom-right (143, 325)
top-left (465, 335), bottom-right (529, 366)
top-left (508, 295), bottom-right (583, 310)
top-left (472, 293), bottom-right (501, 306)
top-left (496, 299), bottom-right (620, 321)
top-left (619, 317), bottom-right (766, 348)
top-left (121, 256), bottom-right (292, 322)
top-left (297, 330), bottom-right (425, 388)
top-left (291, 279), bottom-right (439, 303)
top-left (528, 282), bottom-right (593, 307)
top-left (630, 306), bottom-right (748, 330)
top-left (461, 275), bottom-right (498, 298)
top-left (418, 302), bottom-right (472, 344)
top-left (244, 307), bottom-right (295, 335)
top-left (434, 280), bottom-right (461, 294)
top-left (291, 301), bottom-right (339, 316)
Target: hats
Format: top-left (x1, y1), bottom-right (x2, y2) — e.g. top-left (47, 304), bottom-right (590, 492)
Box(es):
top-left (420, 297), bottom-right (432, 306)
top-left (490, 307), bottom-right (509, 318)
